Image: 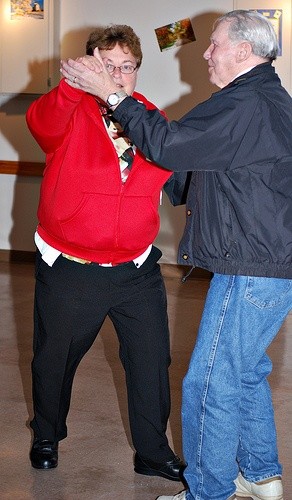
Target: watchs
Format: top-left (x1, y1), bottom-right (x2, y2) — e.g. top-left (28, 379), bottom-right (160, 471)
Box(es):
top-left (105, 90), bottom-right (128, 109)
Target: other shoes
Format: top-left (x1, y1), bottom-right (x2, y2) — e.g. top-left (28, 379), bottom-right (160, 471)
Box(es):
top-left (233, 472), bottom-right (284, 500)
top-left (157, 490), bottom-right (237, 500)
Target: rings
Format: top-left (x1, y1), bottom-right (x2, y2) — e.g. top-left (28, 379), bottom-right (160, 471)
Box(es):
top-left (72, 77), bottom-right (77, 83)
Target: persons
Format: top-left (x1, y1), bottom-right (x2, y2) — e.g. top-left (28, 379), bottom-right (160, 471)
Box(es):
top-left (60, 10), bottom-right (292, 500)
top-left (26, 24), bottom-right (185, 481)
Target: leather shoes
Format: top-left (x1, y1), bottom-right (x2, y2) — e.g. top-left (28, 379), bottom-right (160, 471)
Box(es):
top-left (134, 453), bottom-right (186, 482)
top-left (30, 432), bottom-right (59, 470)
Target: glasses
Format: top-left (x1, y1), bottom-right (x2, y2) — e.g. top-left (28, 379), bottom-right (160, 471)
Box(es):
top-left (106, 64), bottom-right (137, 74)
